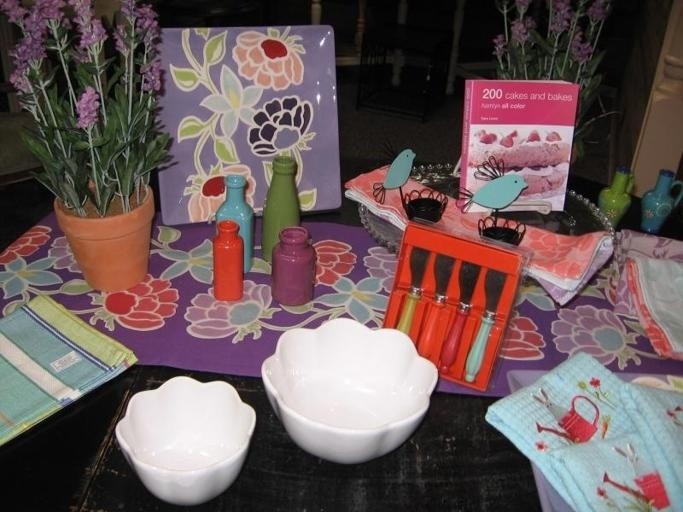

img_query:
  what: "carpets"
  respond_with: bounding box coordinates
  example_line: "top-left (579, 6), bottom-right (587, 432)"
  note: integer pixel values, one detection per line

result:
top-left (0, 208), bottom-right (683, 402)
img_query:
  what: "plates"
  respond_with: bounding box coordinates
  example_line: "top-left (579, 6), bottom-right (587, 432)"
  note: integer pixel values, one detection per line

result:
top-left (361, 160), bottom-right (616, 291)
top-left (145, 24), bottom-right (342, 228)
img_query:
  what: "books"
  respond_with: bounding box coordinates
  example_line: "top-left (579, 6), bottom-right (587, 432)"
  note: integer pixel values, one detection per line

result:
top-left (459, 79), bottom-right (579, 211)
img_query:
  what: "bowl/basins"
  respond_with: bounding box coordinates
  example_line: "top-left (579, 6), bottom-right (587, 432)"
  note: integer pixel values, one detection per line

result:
top-left (260, 318), bottom-right (439, 466)
top-left (110, 375), bottom-right (257, 507)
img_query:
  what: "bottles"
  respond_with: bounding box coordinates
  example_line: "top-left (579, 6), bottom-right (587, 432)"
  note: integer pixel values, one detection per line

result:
top-left (208, 158), bottom-right (316, 305)
top-left (598, 163), bottom-right (682, 234)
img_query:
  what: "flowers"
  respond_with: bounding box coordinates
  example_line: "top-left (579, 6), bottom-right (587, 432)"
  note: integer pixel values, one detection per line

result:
top-left (491, 1), bottom-right (627, 151)
top-left (0, 1), bottom-right (175, 217)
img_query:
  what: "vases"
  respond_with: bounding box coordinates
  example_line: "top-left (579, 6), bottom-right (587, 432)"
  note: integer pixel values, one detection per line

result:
top-left (51, 178), bottom-right (157, 293)
